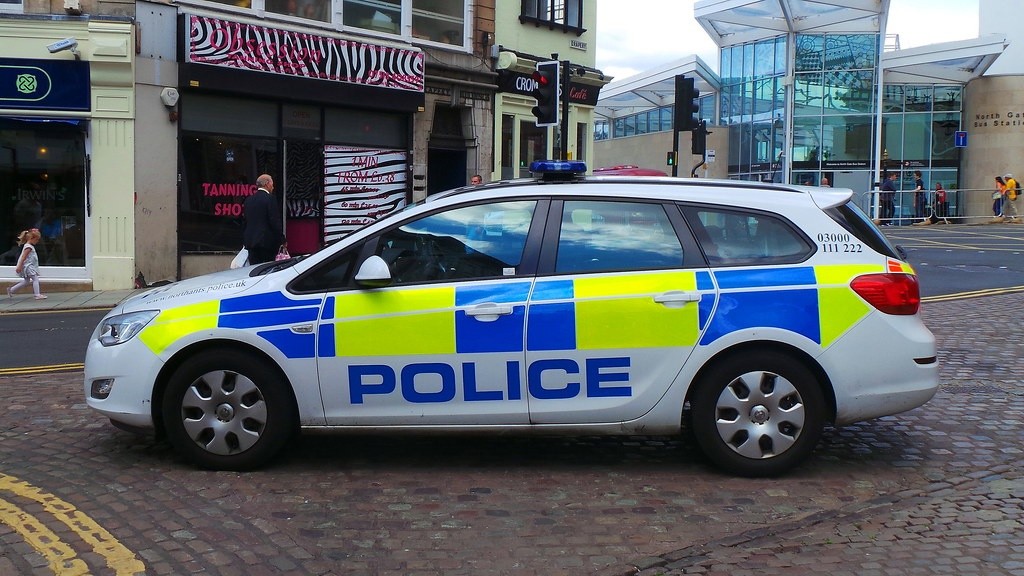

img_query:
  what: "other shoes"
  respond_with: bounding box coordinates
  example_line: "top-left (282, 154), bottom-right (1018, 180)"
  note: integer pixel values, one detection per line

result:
top-left (35, 295), bottom-right (47, 300)
top-left (7, 287), bottom-right (13, 298)
top-left (886, 222), bottom-right (894, 226)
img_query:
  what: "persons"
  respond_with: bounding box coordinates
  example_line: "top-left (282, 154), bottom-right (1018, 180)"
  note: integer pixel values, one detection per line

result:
top-left (880, 173), bottom-right (897, 226)
top-left (992, 174), bottom-right (1018, 218)
top-left (932, 183), bottom-right (946, 222)
top-left (241, 174), bottom-right (288, 265)
top-left (0, 184), bottom-right (67, 263)
top-left (7, 229), bottom-right (47, 299)
top-left (471, 175), bottom-right (482, 185)
top-left (910, 171), bottom-right (926, 222)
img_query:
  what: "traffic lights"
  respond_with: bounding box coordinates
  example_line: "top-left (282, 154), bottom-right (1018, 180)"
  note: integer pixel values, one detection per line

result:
top-left (666, 151), bottom-right (677, 166)
top-left (679, 77), bottom-right (699, 132)
top-left (531, 61), bottom-right (559, 127)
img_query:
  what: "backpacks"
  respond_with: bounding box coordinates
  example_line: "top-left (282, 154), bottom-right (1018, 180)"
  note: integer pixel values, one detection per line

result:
top-left (1014, 179), bottom-right (1022, 195)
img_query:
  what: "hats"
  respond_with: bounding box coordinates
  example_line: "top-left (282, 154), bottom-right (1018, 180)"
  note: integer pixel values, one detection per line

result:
top-left (1003, 174), bottom-right (1012, 179)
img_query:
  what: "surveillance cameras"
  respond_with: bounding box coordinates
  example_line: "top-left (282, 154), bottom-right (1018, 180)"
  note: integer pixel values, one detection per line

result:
top-left (46, 37), bottom-right (77, 54)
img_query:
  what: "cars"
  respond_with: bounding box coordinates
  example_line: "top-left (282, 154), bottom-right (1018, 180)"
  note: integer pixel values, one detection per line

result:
top-left (84, 159), bottom-right (941, 479)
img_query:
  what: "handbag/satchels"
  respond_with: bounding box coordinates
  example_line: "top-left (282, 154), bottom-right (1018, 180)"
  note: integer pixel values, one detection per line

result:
top-left (230, 246), bottom-right (250, 269)
top-left (993, 191), bottom-right (1002, 199)
top-left (275, 245), bottom-right (291, 261)
